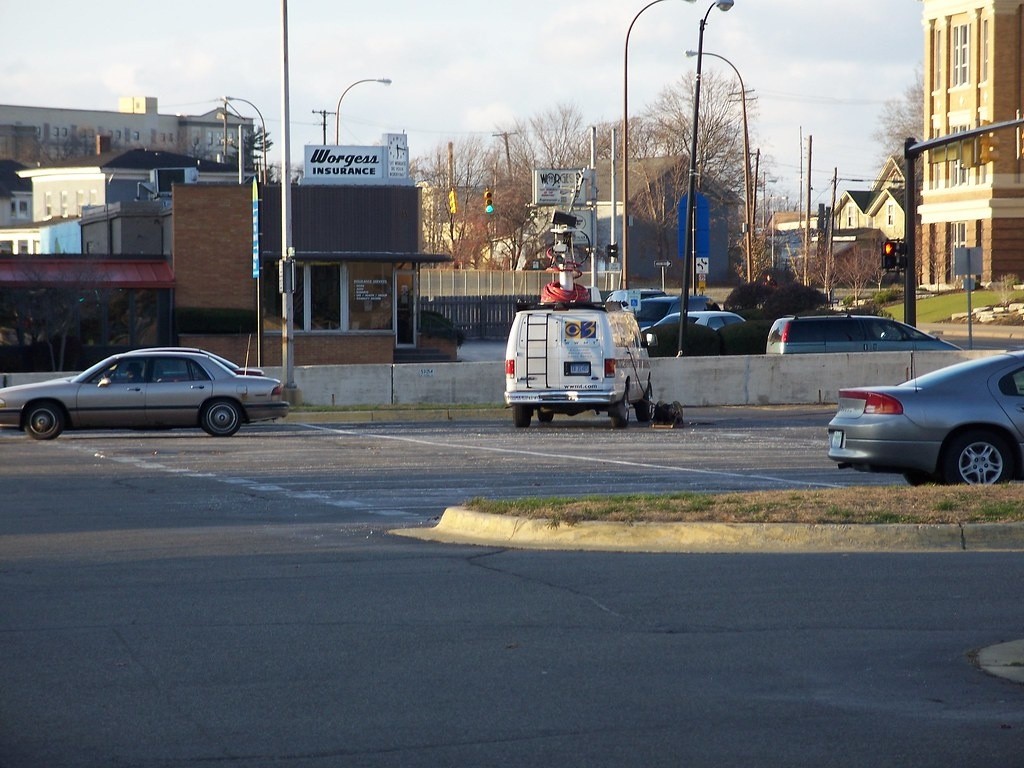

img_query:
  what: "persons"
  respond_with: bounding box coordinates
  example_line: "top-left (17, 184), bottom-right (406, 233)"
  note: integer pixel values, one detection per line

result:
top-left (704, 299), bottom-right (722, 311)
top-left (126, 363), bottom-right (144, 382)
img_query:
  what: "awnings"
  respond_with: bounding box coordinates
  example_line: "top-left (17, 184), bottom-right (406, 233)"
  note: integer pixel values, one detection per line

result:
top-left (261, 251), bottom-right (455, 263)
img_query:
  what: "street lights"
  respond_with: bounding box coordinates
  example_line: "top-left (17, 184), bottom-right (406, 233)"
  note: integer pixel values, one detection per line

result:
top-left (221, 95), bottom-right (266, 186)
top-left (683, 49), bottom-right (754, 285)
top-left (621, 0), bottom-right (699, 289)
top-left (676, 0), bottom-right (737, 356)
top-left (335, 76), bottom-right (393, 145)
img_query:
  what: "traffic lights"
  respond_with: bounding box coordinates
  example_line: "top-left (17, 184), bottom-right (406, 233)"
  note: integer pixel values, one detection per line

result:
top-left (449, 190), bottom-right (459, 213)
top-left (881, 241), bottom-right (897, 271)
top-left (485, 191), bottom-right (493, 214)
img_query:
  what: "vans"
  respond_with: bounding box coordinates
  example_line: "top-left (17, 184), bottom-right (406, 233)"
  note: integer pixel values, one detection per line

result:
top-left (603, 288), bottom-right (668, 310)
top-left (504, 303), bottom-right (656, 428)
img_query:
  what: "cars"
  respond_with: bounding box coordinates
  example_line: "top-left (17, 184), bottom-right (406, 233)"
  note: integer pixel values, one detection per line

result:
top-left (642, 311), bottom-right (747, 333)
top-left (0, 352), bottom-right (292, 437)
top-left (826, 349), bottom-right (1023, 488)
top-left (122, 347), bottom-right (266, 385)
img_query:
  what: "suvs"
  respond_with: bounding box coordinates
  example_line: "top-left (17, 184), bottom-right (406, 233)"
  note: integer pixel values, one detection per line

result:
top-left (633, 295), bottom-right (721, 331)
top-left (764, 312), bottom-right (966, 354)
